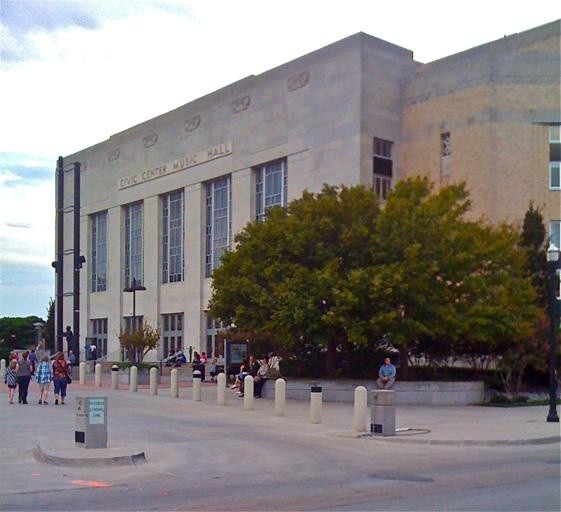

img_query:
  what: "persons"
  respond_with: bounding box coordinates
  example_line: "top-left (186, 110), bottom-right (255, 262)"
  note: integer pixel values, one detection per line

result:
top-left (166, 348), bottom-right (287, 400)
top-left (376, 357), bottom-right (397, 389)
top-left (5, 347), bottom-right (78, 406)
top-left (57, 325), bottom-right (74, 353)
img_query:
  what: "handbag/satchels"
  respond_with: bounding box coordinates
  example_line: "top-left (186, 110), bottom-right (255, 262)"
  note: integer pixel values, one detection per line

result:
top-left (64, 371), bottom-right (71, 384)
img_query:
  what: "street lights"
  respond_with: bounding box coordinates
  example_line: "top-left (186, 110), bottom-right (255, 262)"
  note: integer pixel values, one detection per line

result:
top-left (545, 244), bottom-right (561, 422)
top-left (1, 334), bottom-right (17, 367)
top-left (123, 275), bottom-right (148, 337)
